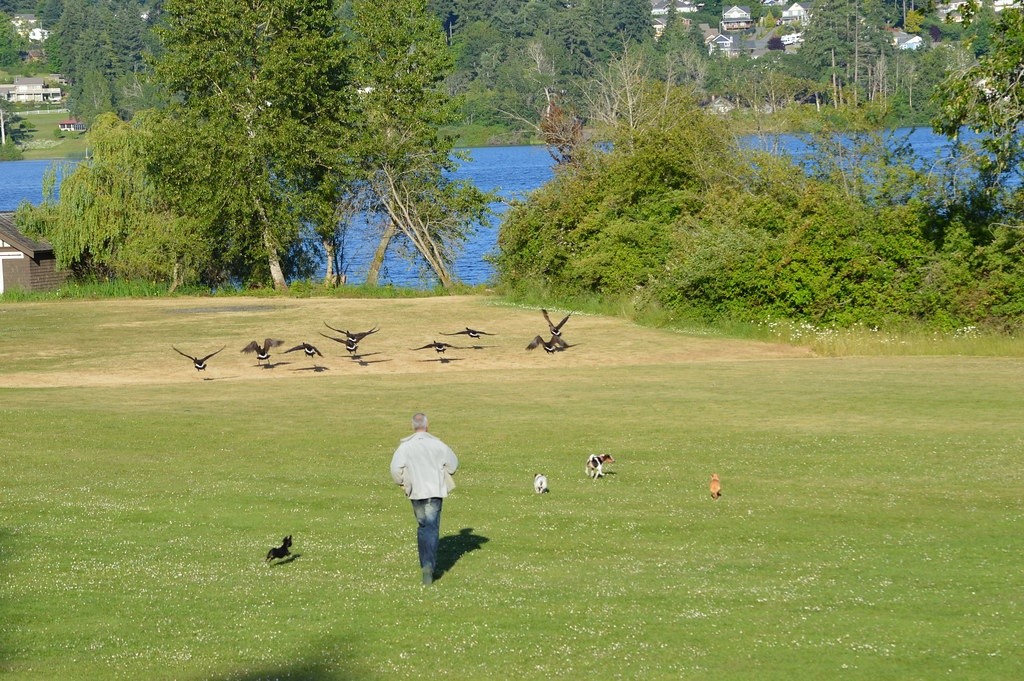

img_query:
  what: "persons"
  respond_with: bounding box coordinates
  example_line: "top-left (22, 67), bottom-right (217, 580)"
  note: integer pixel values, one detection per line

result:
top-left (389, 413), bottom-right (459, 585)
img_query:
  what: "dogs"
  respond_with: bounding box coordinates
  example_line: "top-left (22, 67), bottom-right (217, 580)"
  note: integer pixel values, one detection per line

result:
top-left (534, 474), bottom-right (547, 493)
top-left (585, 454), bottom-right (615, 479)
top-left (710, 473), bottom-right (721, 499)
top-left (265, 534), bottom-right (292, 564)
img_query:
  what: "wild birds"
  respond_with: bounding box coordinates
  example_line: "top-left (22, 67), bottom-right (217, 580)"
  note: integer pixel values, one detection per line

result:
top-left (319, 322), bottom-right (381, 353)
top-left (440, 328), bottom-right (498, 339)
top-left (542, 309), bottom-right (573, 337)
top-left (241, 338), bottom-right (284, 366)
top-left (410, 340), bottom-right (463, 354)
top-left (526, 336), bottom-right (567, 354)
top-left (279, 343), bottom-right (324, 359)
top-left (171, 346), bottom-right (227, 370)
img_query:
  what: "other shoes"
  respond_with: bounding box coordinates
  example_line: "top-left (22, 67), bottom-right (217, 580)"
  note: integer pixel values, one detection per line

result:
top-left (422, 567), bottom-right (433, 585)
